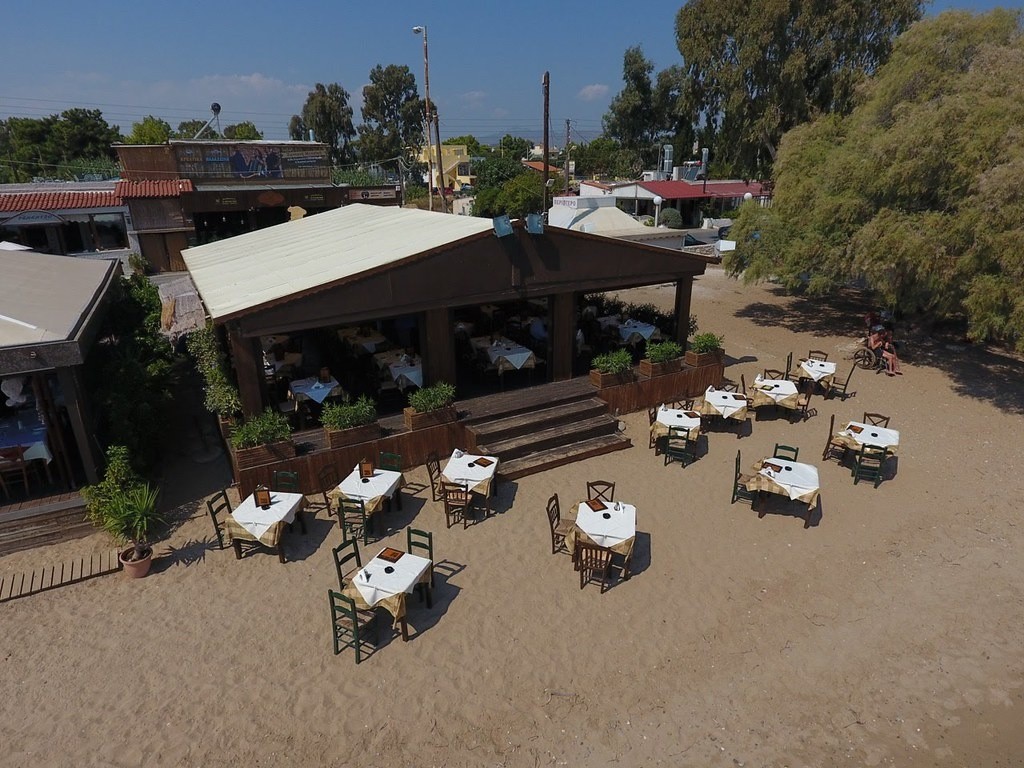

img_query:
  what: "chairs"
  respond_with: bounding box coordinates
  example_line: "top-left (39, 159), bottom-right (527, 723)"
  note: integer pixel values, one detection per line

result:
top-left (204, 348), bottom-right (901, 664)
top-left (265, 365), bottom-right (279, 386)
top-left (270, 394), bottom-right (300, 428)
top-left (0, 443), bottom-right (34, 500)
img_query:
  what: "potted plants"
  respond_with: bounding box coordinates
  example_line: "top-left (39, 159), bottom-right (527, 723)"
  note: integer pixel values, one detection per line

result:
top-left (79, 382), bottom-right (459, 579)
top-left (686, 334), bottom-right (724, 364)
top-left (640, 342), bottom-right (681, 376)
top-left (590, 350), bottom-right (635, 388)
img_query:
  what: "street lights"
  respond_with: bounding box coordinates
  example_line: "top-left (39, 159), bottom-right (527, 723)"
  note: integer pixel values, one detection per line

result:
top-left (653, 195), bottom-right (662, 227)
top-left (412, 24), bottom-right (435, 212)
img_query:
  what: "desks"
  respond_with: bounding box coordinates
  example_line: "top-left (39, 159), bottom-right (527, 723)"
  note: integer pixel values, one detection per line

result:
top-left (656, 407), bottom-right (702, 462)
top-left (703, 388), bottom-right (746, 439)
top-left (0, 412), bottom-right (55, 485)
top-left (754, 380), bottom-right (798, 423)
top-left (846, 422), bottom-right (900, 477)
top-left (352, 547), bottom-right (434, 642)
top-left (574, 500), bottom-right (637, 572)
top-left (445, 451), bottom-right (498, 515)
top-left (329, 467), bottom-right (404, 533)
top-left (797, 357), bottom-right (837, 397)
top-left (264, 299), bottom-right (663, 411)
top-left (224, 489), bottom-right (309, 563)
top-left (755, 456), bottom-right (821, 531)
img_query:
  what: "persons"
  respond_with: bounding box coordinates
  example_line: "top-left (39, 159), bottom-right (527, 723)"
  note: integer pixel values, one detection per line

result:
top-left (698, 201), bottom-right (704, 221)
top-left (229, 144), bottom-right (284, 179)
top-left (863, 307), bottom-right (902, 377)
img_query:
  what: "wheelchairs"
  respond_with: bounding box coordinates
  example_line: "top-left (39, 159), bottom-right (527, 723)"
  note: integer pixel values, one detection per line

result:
top-left (853, 337), bottom-right (895, 376)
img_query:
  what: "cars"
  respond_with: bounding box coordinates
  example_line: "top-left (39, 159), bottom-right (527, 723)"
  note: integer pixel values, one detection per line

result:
top-left (461, 183), bottom-right (475, 190)
